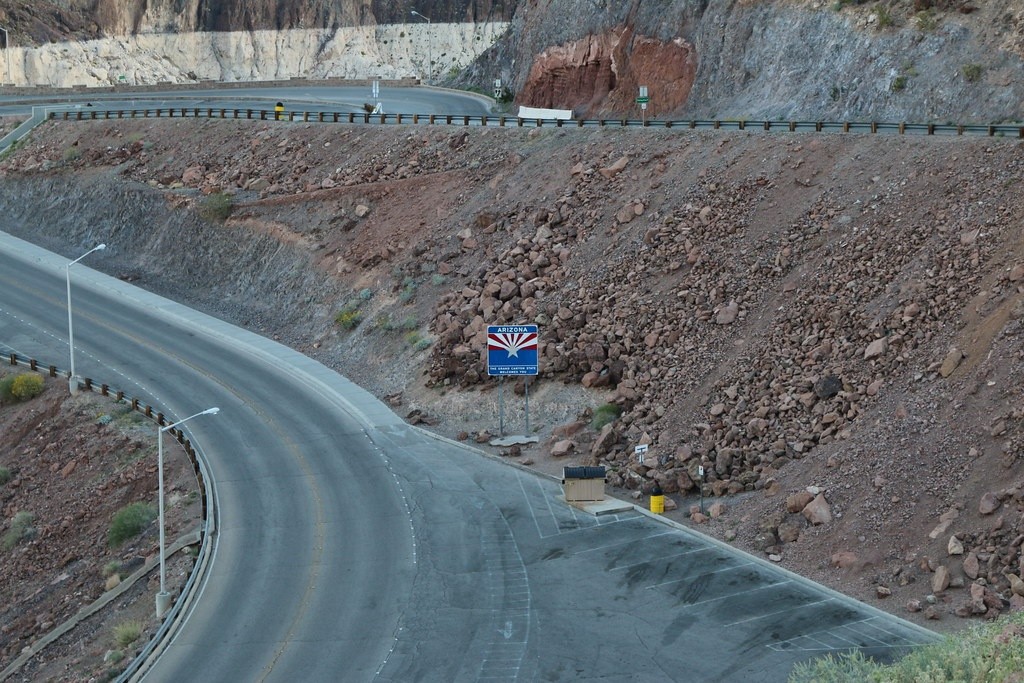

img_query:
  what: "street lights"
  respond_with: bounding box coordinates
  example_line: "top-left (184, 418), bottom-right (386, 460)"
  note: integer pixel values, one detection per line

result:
top-left (0, 27), bottom-right (10, 85)
top-left (412, 11), bottom-right (432, 80)
top-left (65, 240), bottom-right (107, 396)
top-left (153, 405), bottom-right (223, 616)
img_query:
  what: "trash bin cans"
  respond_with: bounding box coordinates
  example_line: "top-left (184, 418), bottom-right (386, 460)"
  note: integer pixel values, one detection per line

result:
top-left (560, 466), bottom-right (608, 502)
top-left (650, 490), bottom-right (665, 514)
